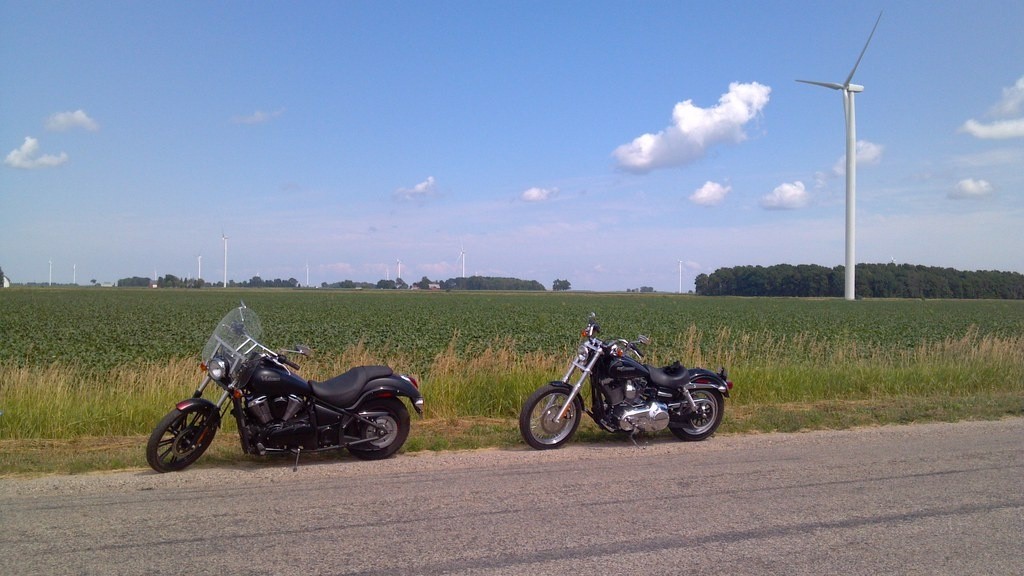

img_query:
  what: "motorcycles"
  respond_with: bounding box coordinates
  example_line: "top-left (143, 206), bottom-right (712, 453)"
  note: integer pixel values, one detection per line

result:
top-left (146, 300), bottom-right (425, 474)
top-left (519, 312), bottom-right (735, 451)
